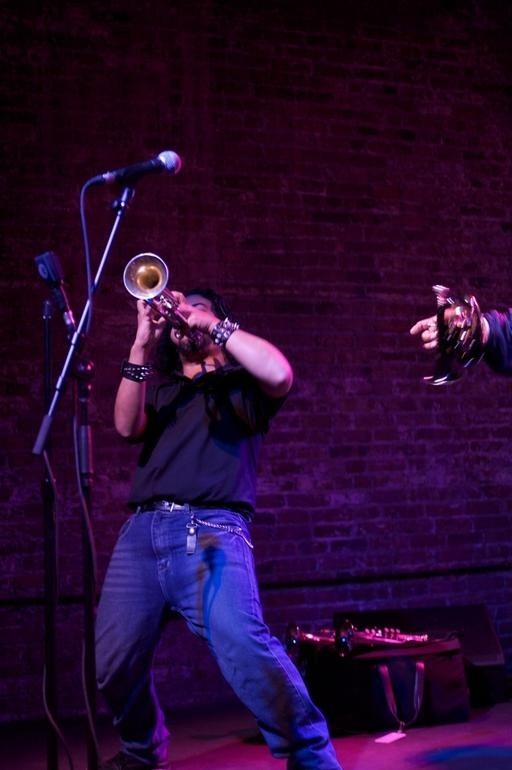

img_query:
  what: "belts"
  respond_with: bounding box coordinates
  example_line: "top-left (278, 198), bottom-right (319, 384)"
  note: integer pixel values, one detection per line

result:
top-left (155, 500), bottom-right (198, 512)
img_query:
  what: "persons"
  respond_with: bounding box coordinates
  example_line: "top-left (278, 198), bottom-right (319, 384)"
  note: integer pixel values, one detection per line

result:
top-left (89, 286), bottom-right (345, 770)
top-left (409, 280), bottom-right (511, 379)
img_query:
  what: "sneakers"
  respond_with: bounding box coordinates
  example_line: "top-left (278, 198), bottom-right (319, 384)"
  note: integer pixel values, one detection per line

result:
top-left (102, 750), bottom-right (170, 770)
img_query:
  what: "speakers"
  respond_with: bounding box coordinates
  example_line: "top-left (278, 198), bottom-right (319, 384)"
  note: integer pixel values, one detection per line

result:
top-left (333, 603), bottom-right (505, 705)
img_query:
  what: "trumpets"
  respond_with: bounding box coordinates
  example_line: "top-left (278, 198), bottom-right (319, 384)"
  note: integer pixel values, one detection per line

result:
top-left (285, 619), bottom-right (429, 659)
top-left (124, 251), bottom-right (198, 343)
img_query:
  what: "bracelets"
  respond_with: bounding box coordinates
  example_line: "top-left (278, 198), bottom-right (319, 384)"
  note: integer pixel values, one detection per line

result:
top-left (121, 361), bottom-right (150, 387)
top-left (207, 316), bottom-right (241, 348)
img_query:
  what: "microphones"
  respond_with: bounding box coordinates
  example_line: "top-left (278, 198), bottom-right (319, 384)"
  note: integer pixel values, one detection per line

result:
top-left (98, 150), bottom-right (184, 185)
top-left (34, 251), bottom-right (75, 338)
